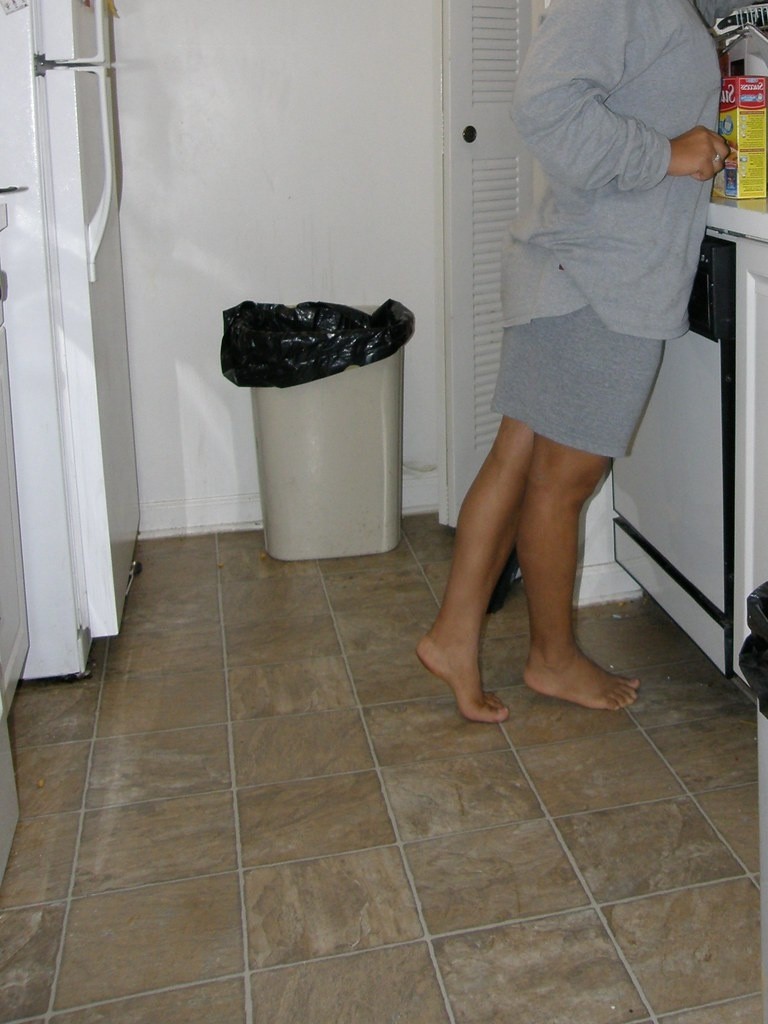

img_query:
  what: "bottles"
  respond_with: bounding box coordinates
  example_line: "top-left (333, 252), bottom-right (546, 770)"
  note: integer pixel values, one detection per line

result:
top-left (723, 162), bottom-right (737, 197)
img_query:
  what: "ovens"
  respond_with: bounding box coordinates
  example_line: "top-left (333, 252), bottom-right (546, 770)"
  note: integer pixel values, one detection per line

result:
top-left (611, 238), bottom-right (736, 679)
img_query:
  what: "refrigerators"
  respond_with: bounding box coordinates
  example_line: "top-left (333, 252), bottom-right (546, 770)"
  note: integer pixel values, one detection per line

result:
top-left (0, 1), bottom-right (141, 679)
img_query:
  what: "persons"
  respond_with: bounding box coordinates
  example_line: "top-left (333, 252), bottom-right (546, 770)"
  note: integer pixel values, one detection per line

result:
top-left (413, 0), bottom-right (762, 724)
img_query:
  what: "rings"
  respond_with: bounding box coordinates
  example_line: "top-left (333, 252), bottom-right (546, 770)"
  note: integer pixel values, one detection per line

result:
top-left (712, 154), bottom-right (720, 163)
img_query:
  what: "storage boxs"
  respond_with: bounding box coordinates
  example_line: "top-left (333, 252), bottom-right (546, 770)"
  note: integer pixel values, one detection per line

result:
top-left (706, 75), bottom-right (766, 200)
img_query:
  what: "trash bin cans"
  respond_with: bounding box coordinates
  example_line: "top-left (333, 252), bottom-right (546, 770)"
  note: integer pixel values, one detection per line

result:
top-left (746, 582), bottom-right (767, 978)
top-left (250, 304), bottom-right (404, 560)
